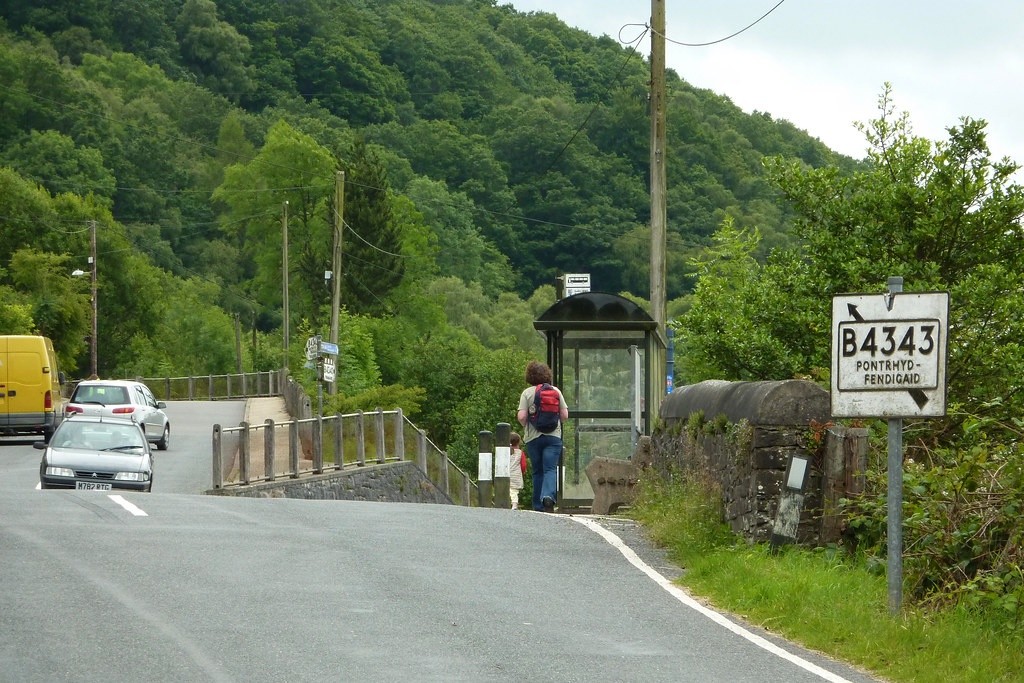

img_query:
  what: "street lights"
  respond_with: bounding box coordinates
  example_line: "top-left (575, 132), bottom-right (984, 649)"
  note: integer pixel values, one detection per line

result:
top-left (71, 219), bottom-right (98, 375)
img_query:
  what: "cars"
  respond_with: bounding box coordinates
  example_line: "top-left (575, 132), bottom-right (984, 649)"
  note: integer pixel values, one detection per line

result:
top-left (33, 410), bottom-right (154, 493)
top-left (66, 380), bottom-right (171, 451)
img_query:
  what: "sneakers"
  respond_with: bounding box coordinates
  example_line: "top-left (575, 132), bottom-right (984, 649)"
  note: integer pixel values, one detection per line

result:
top-left (512, 503), bottom-right (518, 510)
top-left (543, 497), bottom-right (554, 512)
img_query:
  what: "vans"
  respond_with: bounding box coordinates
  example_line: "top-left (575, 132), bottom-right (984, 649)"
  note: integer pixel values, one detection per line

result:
top-left (0, 335), bottom-right (64, 445)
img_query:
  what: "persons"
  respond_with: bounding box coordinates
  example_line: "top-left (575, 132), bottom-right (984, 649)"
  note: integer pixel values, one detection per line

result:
top-left (509, 431), bottom-right (527, 511)
top-left (105, 431), bottom-right (128, 450)
top-left (63, 427), bottom-right (94, 450)
top-left (518, 362), bottom-right (569, 512)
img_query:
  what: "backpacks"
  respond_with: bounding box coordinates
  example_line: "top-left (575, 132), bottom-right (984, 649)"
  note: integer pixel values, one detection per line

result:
top-left (528, 384), bottom-right (560, 433)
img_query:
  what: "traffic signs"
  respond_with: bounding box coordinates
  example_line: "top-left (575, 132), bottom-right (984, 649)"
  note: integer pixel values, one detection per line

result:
top-left (320, 341), bottom-right (339, 355)
top-left (831, 291), bottom-right (951, 419)
top-left (323, 357), bottom-right (336, 382)
top-left (303, 337), bottom-right (319, 361)
